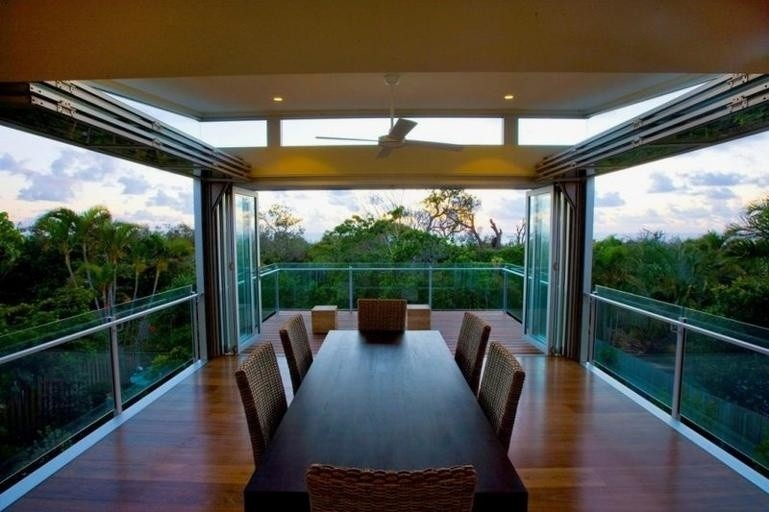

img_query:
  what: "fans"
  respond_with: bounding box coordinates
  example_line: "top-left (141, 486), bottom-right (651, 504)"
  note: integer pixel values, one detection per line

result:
top-left (309, 71), bottom-right (467, 162)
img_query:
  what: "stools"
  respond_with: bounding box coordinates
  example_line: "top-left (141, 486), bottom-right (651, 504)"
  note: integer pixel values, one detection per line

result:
top-left (405, 302), bottom-right (431, 330)
top-left (310, 304), bottom-right (338, 336)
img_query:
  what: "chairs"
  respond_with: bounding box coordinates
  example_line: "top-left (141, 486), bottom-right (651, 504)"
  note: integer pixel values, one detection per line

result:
top-left (475, 339), bottom-right (526, 456)
top-left (232, 339), bottom-right (289, 469)
top-left (355, 297), bottom-right (407, 333)
top-left (302, 460), bottom-right (479, 511)
top-left (277, 310), bottom-right (314, 399)
top-left (453, 310), bottom-right (492, 398)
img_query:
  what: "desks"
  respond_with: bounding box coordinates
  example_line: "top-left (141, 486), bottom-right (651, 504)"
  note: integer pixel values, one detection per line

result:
top-left (241, 328), bottom-right (530, 512)
